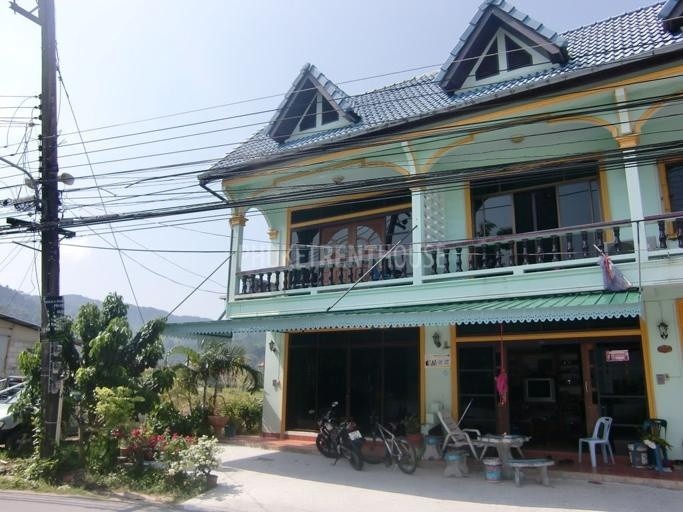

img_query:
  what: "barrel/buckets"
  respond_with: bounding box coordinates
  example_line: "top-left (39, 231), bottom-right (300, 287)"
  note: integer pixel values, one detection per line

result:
top-left (483, 457), bottom-right (503, 480)
top-left (627, 443), bottom-right (648, 469)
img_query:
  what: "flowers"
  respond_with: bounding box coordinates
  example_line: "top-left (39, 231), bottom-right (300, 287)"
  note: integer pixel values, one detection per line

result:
top-left (110, 428), bottom-right (193, 462)
top-left (178, 434), bottom-right (225, 473)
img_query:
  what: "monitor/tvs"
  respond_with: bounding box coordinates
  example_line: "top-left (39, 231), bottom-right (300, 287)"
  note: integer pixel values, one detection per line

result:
top-left (522, 378), bottom-right (556, 402)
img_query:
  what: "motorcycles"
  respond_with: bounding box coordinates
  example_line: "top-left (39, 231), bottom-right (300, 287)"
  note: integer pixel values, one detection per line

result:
top-left (306, 397), bottom-right (366, 472)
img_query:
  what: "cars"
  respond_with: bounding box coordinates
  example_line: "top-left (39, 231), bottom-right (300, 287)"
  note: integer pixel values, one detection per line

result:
top-left (0, 385), bottom-right (34, 448)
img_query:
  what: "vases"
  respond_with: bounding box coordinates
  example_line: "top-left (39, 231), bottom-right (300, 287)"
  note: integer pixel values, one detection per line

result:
top-left (199, 473), bottom-right (218, 489)
top-left (118, 448), bottom-right (164, 462)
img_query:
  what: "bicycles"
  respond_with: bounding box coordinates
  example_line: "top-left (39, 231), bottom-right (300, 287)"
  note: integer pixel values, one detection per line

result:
top-left (361, 409), bottom-right (418, 474)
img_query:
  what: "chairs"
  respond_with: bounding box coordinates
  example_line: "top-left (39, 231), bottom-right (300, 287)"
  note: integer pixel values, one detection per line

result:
top-left (437, 408), bottom-right (531, 480)
top-left (578, 416), bottom-right (616, 467)
top-left (632, 419), bottom-right (668, 472)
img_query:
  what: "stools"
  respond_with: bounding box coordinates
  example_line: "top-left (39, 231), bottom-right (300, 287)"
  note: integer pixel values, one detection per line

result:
top-left (506, 457), bottom-right (554, 487)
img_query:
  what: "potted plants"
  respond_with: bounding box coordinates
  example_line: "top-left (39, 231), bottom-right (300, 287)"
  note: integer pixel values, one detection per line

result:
top-left (400, 412), bottom-right (423, 448)
